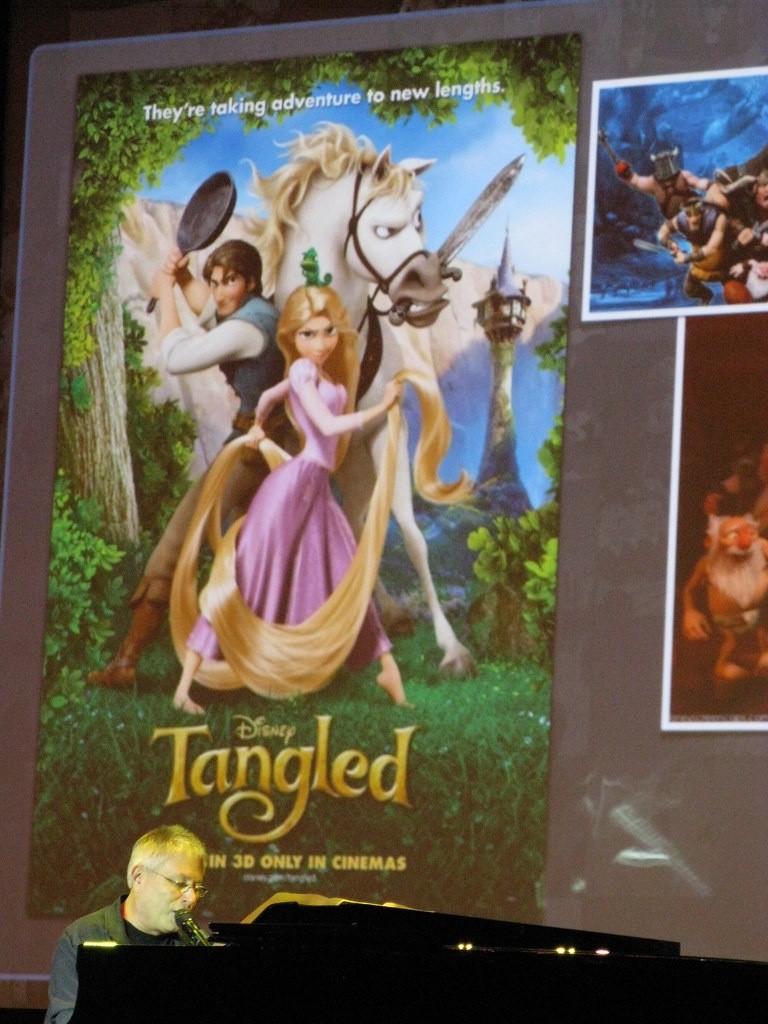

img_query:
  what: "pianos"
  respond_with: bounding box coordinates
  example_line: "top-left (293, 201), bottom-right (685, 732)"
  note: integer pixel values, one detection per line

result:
top-left (65, 901), bottom-right (768, 1024)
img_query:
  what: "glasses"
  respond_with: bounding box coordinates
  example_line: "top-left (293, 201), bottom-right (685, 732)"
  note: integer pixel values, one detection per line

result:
top-left (144, 867), bottom-right (207, 898)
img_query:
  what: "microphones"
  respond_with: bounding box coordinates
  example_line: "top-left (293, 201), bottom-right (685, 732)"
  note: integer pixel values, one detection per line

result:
top-left (174, 909), bottom-right (212, 946)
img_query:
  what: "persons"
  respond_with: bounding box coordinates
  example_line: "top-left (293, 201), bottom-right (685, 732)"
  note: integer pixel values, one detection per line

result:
top-left (44, 823), bottom-right (210, 1024)
